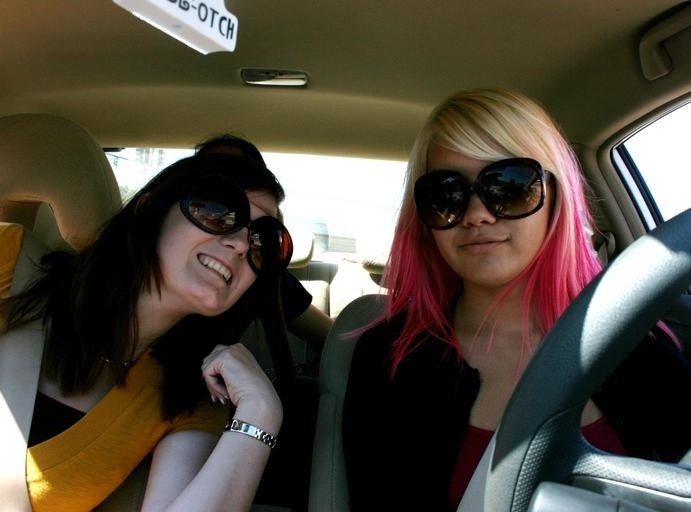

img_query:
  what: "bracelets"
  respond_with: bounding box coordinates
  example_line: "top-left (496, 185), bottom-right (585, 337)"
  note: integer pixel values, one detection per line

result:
top-left (224, 416), bottom-right (277, 449)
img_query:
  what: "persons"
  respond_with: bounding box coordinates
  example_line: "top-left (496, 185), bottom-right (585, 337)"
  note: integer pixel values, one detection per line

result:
top-left (0, 135), bottom-right (286, 511)
top-left (188, 136), bottom-right (333, 341)
top-left (344, 83), bottom-right (689, 512)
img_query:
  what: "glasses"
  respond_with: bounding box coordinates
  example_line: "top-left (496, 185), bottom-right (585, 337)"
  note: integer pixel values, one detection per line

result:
top-left (411, 157), bottom-right (548, 231)
top-left (178, 179), bottom-right (293, 281)
